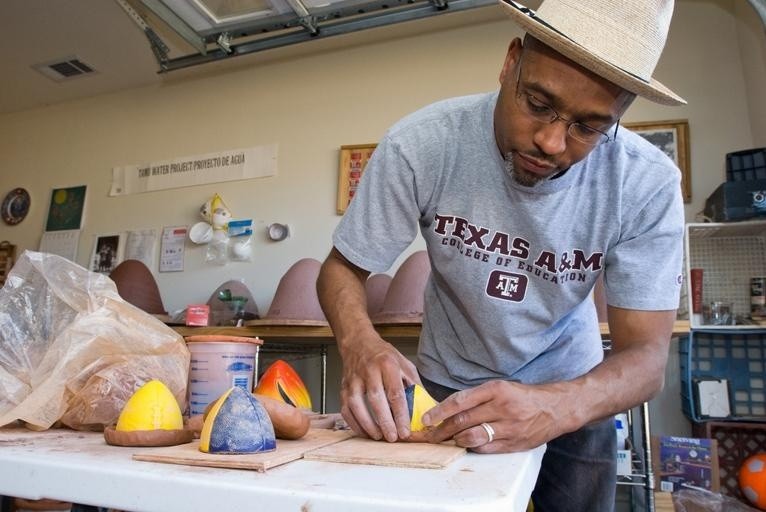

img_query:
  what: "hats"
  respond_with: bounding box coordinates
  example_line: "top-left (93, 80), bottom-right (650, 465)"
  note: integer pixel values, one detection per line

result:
top-left (498, 0), bottom-right (688, 108)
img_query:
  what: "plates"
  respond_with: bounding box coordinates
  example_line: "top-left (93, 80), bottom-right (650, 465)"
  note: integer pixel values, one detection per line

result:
top-left (1, 187), bottom-right (30, 225)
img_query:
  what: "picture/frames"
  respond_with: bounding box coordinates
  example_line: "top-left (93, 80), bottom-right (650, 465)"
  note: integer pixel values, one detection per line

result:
top-left (619, 118), bottom-right (692, 204)
top-left (337, 143), bottom-right (377, 215)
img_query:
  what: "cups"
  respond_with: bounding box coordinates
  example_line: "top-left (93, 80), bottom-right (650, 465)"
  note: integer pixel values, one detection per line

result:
top-left (702, 301), bottom-right (734, 325)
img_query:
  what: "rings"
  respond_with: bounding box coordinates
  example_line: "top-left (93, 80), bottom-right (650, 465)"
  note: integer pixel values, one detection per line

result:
top-left (482, 419), bottom-right (498, 442)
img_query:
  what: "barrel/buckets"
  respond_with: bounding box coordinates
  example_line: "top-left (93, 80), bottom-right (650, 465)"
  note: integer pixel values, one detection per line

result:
top-left (186, 340), bottom-right (258, 420)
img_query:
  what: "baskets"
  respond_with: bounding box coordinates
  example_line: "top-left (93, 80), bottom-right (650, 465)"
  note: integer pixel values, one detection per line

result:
top-left (679, 326), bottom-right (766, 422)
top-left (684, 218), bottom-right (762, 329)
top-left (692, 421), bottom-right (765, 503)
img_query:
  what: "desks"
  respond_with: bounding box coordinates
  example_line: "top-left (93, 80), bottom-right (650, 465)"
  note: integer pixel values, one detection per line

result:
top-left (0, 417), bottom-right (547, 512)
top-left (172, 320), bottom-right (692, 512)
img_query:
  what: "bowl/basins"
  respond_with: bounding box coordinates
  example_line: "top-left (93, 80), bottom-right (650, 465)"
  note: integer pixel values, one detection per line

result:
top-left (212, 300), bottom-right (244, 322)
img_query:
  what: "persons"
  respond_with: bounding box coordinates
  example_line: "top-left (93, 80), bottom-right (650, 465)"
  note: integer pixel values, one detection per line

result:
top-left (319, 1), bottom-right (686, 511)
top-left (105, 245), bottom-right (112, 272)
top-left (99, 243), bottom-right (108, 272)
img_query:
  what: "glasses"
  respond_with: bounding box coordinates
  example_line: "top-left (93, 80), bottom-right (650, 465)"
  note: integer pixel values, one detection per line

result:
top-left (514, 58), bottom-right (620, 146)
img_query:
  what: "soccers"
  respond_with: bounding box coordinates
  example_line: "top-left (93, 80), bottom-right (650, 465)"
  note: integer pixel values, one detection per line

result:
top-left (739, 454), bottom-right (766, 510)
